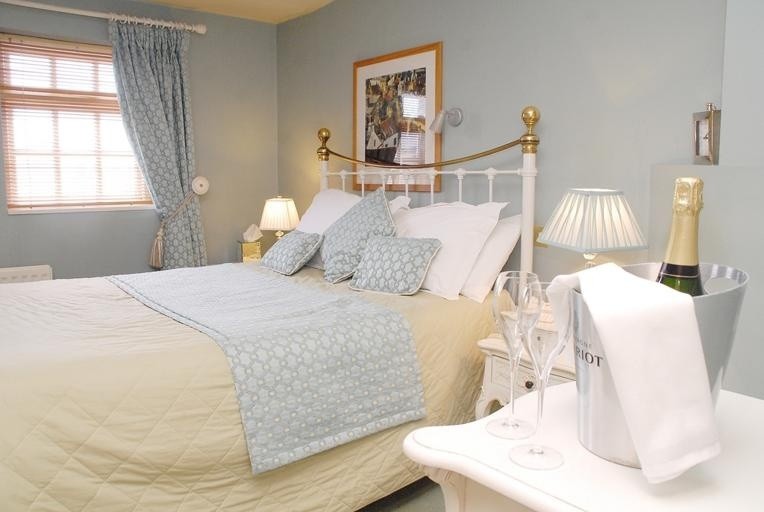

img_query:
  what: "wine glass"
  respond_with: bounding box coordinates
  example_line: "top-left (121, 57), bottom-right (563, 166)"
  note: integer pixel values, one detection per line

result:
top-left (486, 269), bottom-right (572, 470)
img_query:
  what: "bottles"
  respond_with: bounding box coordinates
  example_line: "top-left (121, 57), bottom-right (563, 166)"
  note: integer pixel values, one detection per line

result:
top-left (656, 176), bottom-right (704, 296)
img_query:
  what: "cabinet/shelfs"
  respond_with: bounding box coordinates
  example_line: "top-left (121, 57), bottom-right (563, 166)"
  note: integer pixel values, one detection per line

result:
top-left (402, 381), bottom-right (763, 512)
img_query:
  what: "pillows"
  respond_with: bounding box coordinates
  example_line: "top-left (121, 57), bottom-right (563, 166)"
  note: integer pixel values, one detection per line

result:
top-left (259, 188), bottom-right (522, 305)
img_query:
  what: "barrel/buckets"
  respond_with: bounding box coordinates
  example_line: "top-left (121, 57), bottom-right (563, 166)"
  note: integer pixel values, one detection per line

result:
top-left (571, 263), bottom-right (748, 470)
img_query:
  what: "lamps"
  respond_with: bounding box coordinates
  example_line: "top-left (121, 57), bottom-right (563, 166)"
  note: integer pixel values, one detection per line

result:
top-left (536, 188), bottom-right (651, 272)
top-left (430, 107), bottom-right (462, 135)
top-left (258, 195), bottom-right (301, 240)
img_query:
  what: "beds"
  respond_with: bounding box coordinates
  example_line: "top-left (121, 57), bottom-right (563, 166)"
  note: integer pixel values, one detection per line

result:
top-left (0, 105), bottom-right (540, 512)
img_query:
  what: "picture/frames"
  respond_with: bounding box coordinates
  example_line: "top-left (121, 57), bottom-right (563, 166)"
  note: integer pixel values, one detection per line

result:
top-left (352, 40), bottom-right (444, 192)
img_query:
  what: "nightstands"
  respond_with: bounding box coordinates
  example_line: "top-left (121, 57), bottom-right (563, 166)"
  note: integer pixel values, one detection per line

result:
top-left (474, 303), bottom-right (575, 426)
